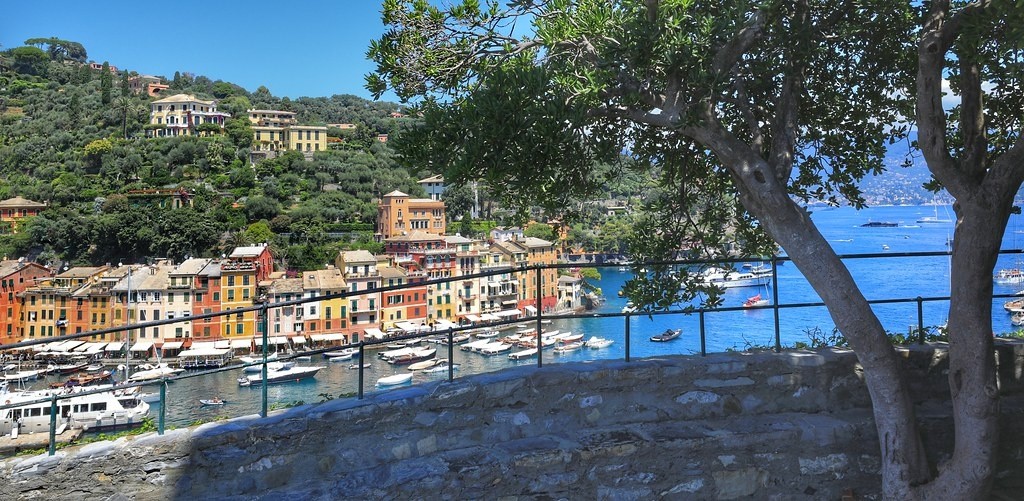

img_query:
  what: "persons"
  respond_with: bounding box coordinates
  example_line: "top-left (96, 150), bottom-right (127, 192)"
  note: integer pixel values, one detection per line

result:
top-left (213, 396), bottom-right (218, 403)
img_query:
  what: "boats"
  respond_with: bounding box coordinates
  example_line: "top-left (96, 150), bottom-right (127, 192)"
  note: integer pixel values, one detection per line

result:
top-left (915, 178), bottom-right (954, 224)
top-left (421, 363), bottom-right (461, 376)
top-left (740, 260), bottom-right (771, 307)
top-left (669, 262), bottom-right (774, 291)
top-left (475, 328), bottom-right (500, 338)
top-left (905, 223), bottom-right (922, 229)
top-left (649, 328), bottom-right (683, 341)
top-left (349, 363), bottom-right (372, 370)
top-left (622, 299), bottom-right (639, 313)
top-left (406, 356), bottom-right (449, 371)
top-left (992, 264), bottom-right (1024, 285)
top-left (1003, 290), bottom-right (1024, 327)
top-left (507, 347), bottom-right (538, 361)
top-left (860, 220), bottom-right (898, 228)
top-left (462, 318), bottom-right (614, 354)
top-left (329, 353), bottom-right (353, 363)
top-left (0, 266), bottom-right (327, 433)
top-left (379, 337), bottom-right (439, 368)
top-left (374, 372), bottom-right (414, 389)
top-left (198, 396), bottom-right (227, 405)
top-left (441, 333), bottom-right (471, 343)
top-left (321, 349), bottom-right (360, 360)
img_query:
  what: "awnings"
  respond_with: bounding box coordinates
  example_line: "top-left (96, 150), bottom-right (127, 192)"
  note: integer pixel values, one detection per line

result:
top-left (310, 333), bottom-right (345, 342)
top-left (130, 340), bottom-right (156, 352)
top-left (161, 341), bottom-right (184, 350)
top-left (364, 328), bottom-right (384, 340)
top-left (10, 337), bottom-right (125, 357)
top-left (465, 309), bottom-right (522, 321)
top-left (488, 281), bottom-right (501, 287)
top-left (189, 338), bottom-right (251, 349)
top-left (254, 335), bottom-right (307, 346)
top-left (502, 299), bottom-right (518, 305)
top-left (394, 321), bottom-right (415, 333)
